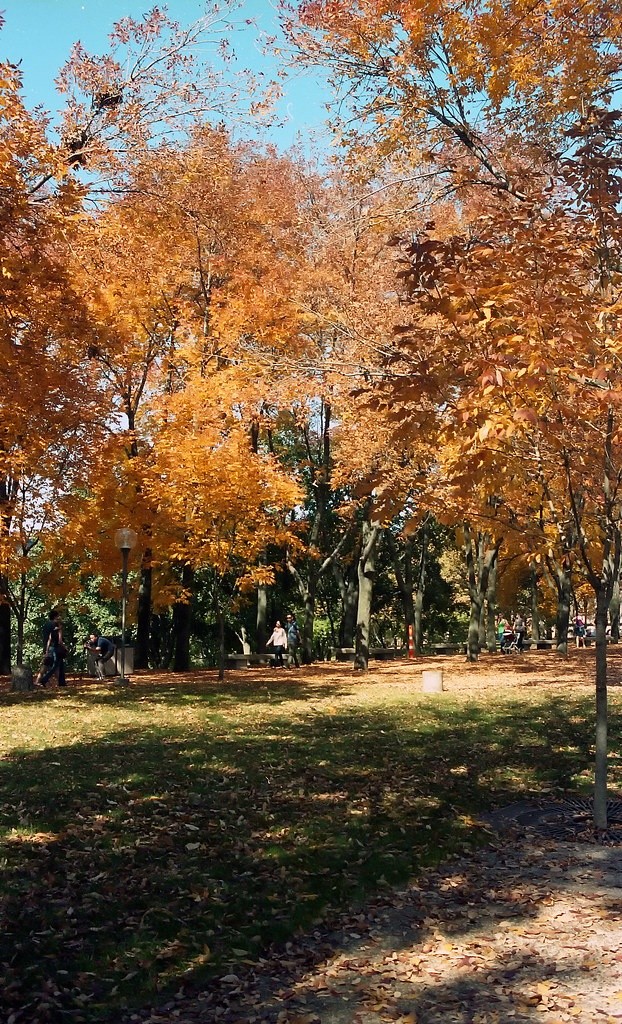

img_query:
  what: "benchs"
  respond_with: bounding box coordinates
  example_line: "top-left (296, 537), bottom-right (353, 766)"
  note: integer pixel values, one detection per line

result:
top-left (585, 636), bottom-right (615, 648)
top-left (431, 642), bottom-right (468, 655)
top-left (225, 654), bottom-right (288, 670)
top-left (335, 648), bottom-right (395, 663)
top-left (521, 639), bottom-right (557, 650)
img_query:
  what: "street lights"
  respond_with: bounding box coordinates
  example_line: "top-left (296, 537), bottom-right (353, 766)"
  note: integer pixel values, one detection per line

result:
top-left (114, 527), bottom-right (137, 688)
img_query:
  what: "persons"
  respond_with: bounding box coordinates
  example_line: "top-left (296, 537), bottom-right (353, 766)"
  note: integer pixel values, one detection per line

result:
top-left (83, 633), bottom-right (115, 681)
top-left (513, 612), bottom-right (526, 652)
top-left (265, 619), bottom-right (288, 668)
top-left (34, 610), bottom-right (59, 686)
top-left (39, 616), bottom-right (67, 687)
top-left (494, 611), bottom-right (516, 653)
top-left (572, 610), bottom-right (587, 649)
top-left (284, 613), bottom-right (303, 669)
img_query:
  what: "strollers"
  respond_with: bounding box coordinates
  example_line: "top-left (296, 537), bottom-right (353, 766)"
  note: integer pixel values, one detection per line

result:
top-left (502, 628), bottom-right (524, 655)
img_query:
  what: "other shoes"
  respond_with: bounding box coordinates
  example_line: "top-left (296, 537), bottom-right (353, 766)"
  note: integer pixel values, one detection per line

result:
top-left (293, 665), bottom-right (300, 668)
top-left (34, 683), bottom-right (47, 689)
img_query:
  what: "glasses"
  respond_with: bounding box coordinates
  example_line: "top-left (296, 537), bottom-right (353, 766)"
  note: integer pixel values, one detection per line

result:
top-left (287, 617), bottom-right (292, 619)
